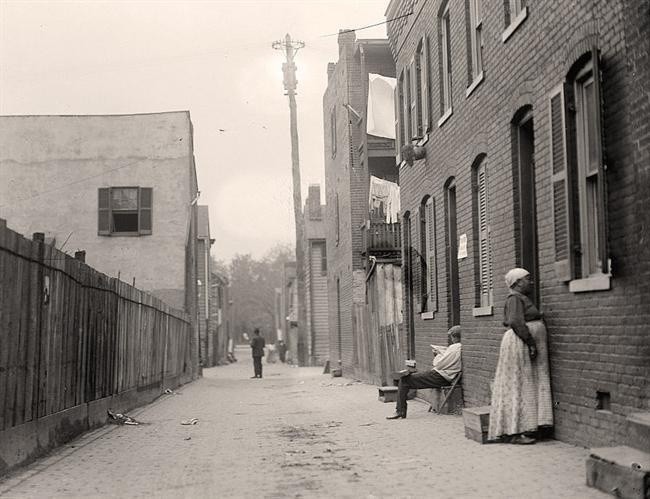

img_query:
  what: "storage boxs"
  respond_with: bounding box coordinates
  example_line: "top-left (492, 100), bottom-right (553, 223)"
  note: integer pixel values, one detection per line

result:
top-left (377, 386), bottom-right (398, 403)
top-left (462, 405), bottom-right (491, 432)
top-left (464, 426), bottom-right (504, 444)
top-left (585, 444), bottom-right (650, 499)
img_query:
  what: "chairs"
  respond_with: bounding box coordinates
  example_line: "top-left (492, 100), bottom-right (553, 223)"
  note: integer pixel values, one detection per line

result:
top-left (427, 371), bottom-right (463, 415)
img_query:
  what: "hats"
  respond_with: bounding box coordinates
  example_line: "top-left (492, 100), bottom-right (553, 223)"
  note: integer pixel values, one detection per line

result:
top-left (504, 267), bottom-right (529, 287)
top-left (446, 325), bottom-right (460, 334)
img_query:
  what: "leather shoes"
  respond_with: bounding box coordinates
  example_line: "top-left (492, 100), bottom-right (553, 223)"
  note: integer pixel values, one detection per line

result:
top-left (512, 437), bottom-right (536, 446)
top-left (386, 411), bottom-right (406, 419)
top-left (389, 369), bottom-right (410, 380)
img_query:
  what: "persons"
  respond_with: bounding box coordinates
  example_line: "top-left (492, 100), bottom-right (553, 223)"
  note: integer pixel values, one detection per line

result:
top-left (486, 268), bottom-right (553, 445)
top-left (247, 327), bottom-right (265, 380)
top-left (386, 324), bottom-right (462, 418)
